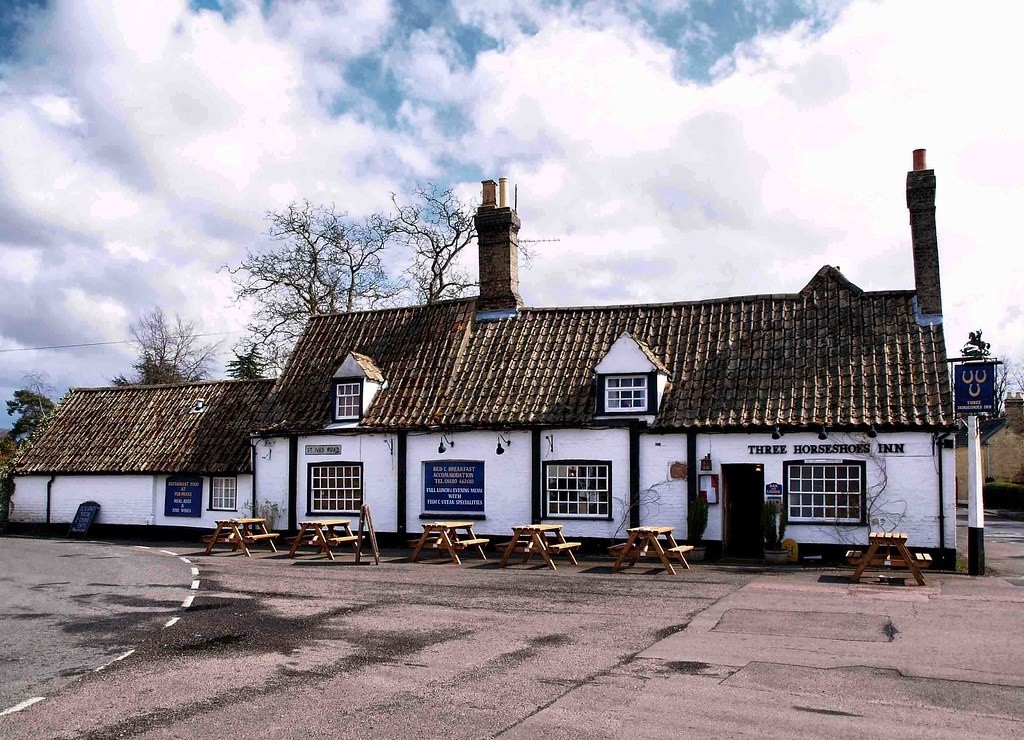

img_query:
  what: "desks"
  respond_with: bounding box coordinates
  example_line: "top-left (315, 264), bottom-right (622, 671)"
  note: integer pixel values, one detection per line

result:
top-left (205, 518), bottom-right (277, 557)
top-left (611, 526), bottom-right (689, 575)
top-left (500, 524), bottom-right (577, 571)
top-left (409, 522), bottom-right (487, 564)
top-left (288, 520), bottom-right (362, 560)
top-left (851, 532), bottom-right (926, 586)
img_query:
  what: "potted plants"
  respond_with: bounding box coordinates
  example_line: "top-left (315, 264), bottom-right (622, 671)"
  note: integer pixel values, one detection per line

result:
top-left (238, 497), bottom-right (285, 547)
top-left (683, 496), bottom-right (709, 562)
top-left (761, 501), bottom-right (789, 564)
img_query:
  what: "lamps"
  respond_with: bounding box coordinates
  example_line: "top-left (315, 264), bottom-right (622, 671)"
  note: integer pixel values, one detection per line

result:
top-left (771, 428), bottom-right (784, 440)
top-left (868, 426), bottom-right (878, 438)
top-left (818, 427), bottom-right (828, 440)
top-left (700, 453), bottom-right (713, 471)
top-left (495, 434), bottom-right (511, 455)
top-left (438, 434), bottom-right (454, 453)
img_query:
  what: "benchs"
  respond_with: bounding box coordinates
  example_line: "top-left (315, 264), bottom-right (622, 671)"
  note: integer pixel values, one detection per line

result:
top-left (846, 550), bottom-right (862, 559)
top-left (607, 543), bottom-right (636, 551)
top-left (496, 541), bottom-right (581, 551)
top-left (202, 532), bottom-right (280, 540)
top-left (916, 552), bottom-right (933, 561)
top-left (408, 537), bottom-right (489, 545)
top-left (668, 545), bottom-right (694, 554)
top-left (285, 534), bottom-right (366, 543)
